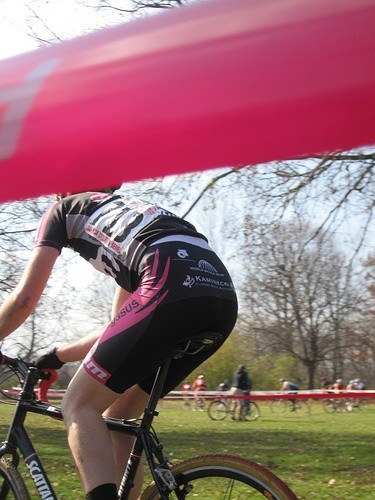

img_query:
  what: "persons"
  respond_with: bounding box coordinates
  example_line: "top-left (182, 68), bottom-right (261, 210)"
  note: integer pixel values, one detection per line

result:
top-left (2, 181), bottom-right (239, 500)
top-left (180, 374), bottom-right (374, 409)
top-left (242, 376), bottom-right (252, 417)
top-left (228, 365), bottom-right (248, 421)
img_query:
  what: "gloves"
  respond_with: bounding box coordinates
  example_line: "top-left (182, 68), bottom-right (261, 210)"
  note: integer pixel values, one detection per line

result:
top-left (35, 347), bottom-right (65, 381)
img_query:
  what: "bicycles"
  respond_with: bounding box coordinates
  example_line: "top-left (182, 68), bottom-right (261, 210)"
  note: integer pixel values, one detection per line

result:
top-left (268, 395), bottom-right (308, 414)
top-left (0, 330), bottom-right (291, 500)
top-left (322, 396), bottom-right (367, 414)
top-left (207, 392), bottom-right (261, 421)
